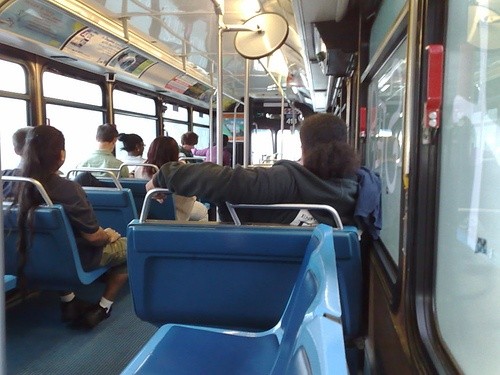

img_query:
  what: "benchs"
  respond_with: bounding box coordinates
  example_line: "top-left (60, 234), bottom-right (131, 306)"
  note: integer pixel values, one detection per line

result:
top-left (0, 176), bottom-right (113, 321)
top-left (119, 223), bottom-right (350, 375)
top-left (125, 188), bottom-right (365, 342)
top-left (119, 223), bottom-right (349, 375)
top-left (66, 170), bottom-right (138, 237)
top-left (69, 163), bottom-right (160, 220)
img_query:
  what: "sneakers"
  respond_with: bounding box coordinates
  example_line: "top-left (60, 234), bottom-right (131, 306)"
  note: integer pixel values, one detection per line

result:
top-left (61, 296), bottom-right (94, 323)
top-left (82, 300), bottom-right (112, 328)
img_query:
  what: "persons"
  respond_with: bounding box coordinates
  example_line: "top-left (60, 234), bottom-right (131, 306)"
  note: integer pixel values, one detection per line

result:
top-left (134, 136), bottom-right (180, 180)
top-left (11, 125), bottom-right (128, 329)
top-left (0, 126), bottom-right (110, 202)
top-left (191, 134), bottom-right (231, 166)
top-left (117, 133), bottom-right (146, 174)
top-left (145, 112), bottom-right (382, 252)
top-left (177, 131), bottom-right (199, 163)
top-left (73, 123), bottom-right (129, 178)
